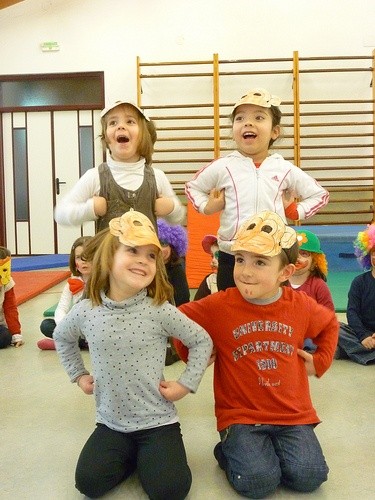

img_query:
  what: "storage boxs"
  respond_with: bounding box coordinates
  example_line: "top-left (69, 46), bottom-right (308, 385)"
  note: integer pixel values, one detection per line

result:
top-left (308, 229), bottom-right (367, 270)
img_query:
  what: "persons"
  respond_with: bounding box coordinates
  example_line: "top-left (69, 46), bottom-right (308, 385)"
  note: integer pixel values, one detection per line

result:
top-left (156, 218), bottom-right (191, 307)
top-left (283, 229), bottom-right (335, 311)
top-left (53, 208), bottom-right (213, 500)
top-left (0, 246), bottom-right (24, 348)
top-left (194, 235), bottom-right (219, 301)
top-left (334, 221), bottom-right (375, 366)
top-left (184, 90), bottom-right (330, 292)
top-left (37, 236), bottom-right (94, 350)
top-left (54, 101), bottom-right (186, 235)
top-left (173, 212), bottom-right (328, 496)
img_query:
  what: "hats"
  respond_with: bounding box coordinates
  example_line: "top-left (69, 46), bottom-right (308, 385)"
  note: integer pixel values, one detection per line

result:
top-left (202, 235), bottom-right (217, 254)
top-left (295, 229), bottom-right (324, 255)
